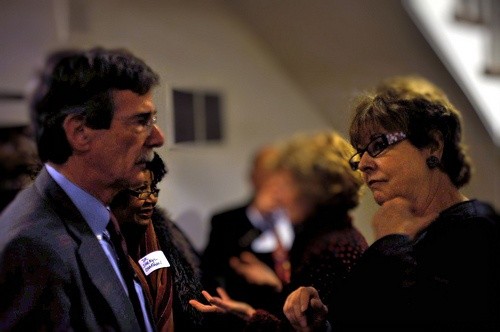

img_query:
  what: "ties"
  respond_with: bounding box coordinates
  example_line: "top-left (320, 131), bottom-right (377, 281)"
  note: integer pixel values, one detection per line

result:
top-left (105, 209), bottom-right (149, 332)
top-left (265, 218), bottom-right (291, 284)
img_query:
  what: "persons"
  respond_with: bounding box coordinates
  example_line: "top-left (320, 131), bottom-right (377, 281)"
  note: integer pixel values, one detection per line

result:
top-left (200, 141), bottom-right (291, 332)
top-left (282, 75), bottom-right (500, 332)
top-left (108, 152), bottom-right (200, 332)
top-left (0, 45), bottom-right (161, 332)
top-left (189, 130), bottom-right (370, 332)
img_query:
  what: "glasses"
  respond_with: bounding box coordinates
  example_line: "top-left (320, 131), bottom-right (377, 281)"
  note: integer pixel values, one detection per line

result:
top-left (348, 129), bottom-right (407, 171)
top-left (124, 187), bottom-right (161, 200)
